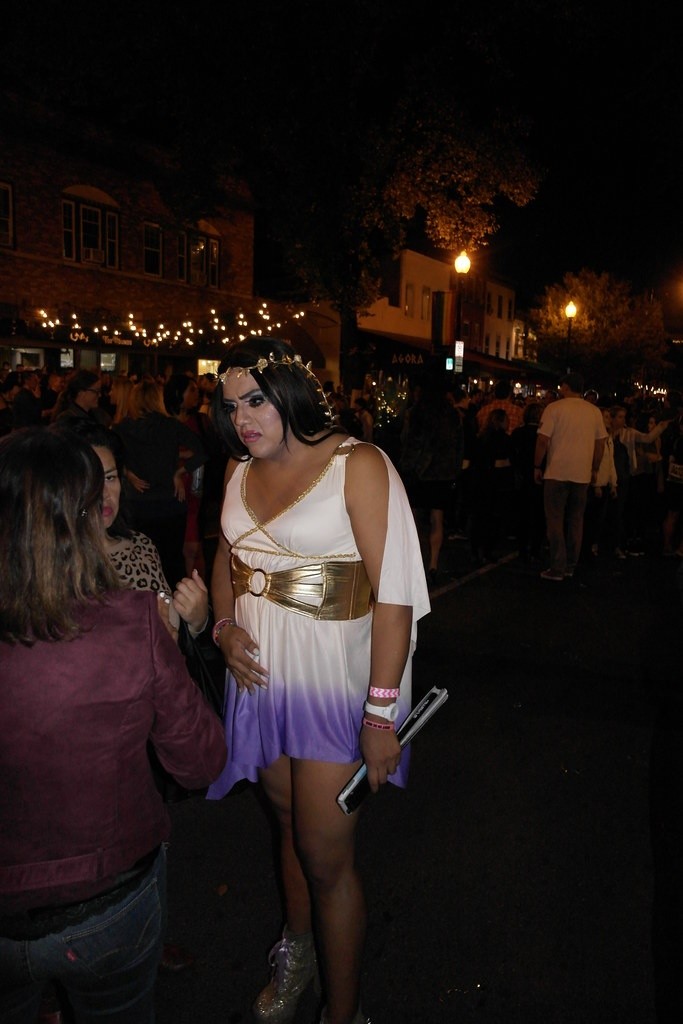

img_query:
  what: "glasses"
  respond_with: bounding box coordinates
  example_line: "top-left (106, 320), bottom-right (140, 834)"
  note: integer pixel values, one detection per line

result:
top-left (84, 388), bottom-right (101, 395)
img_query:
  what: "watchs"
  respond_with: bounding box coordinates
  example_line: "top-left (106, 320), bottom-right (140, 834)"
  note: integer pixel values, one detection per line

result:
top-left (364, 700), bottom-right (400, 722)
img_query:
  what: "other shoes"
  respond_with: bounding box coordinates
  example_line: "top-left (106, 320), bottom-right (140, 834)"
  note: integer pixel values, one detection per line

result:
top-left (540, 569), bottom-right (564, 580)
top-left (564, 569), bottom-right (574, 576)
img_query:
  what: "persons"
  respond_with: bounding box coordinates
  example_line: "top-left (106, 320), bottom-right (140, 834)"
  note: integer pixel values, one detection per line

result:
top-left (206, 335), bottom-right (431, 1024)
top-left (0, 424), bottom-right (228, 1024)
top-left (0, 360), bottom-right (683, 586)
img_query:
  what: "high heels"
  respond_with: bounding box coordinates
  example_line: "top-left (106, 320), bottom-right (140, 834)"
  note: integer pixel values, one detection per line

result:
top-left (253, 924), bottom-right (321, 1023)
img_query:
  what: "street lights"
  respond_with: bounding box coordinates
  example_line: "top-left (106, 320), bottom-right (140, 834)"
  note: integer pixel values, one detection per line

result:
top-left (564, 301), bottom-right (579, 375)
top-left (453, 248), bottom-right (472, 378)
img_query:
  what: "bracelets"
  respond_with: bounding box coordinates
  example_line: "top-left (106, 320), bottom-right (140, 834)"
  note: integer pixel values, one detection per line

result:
top-left (534, 463), bottom-right (542, 471)
top-left (363, 717), bottom-right (395, 731)
top-left (369, 685), bottom-right (400, 699)
top-left (212, 618), bottom-right (236, 647)
top-left (187, 616), bottom-right (209, 639)
